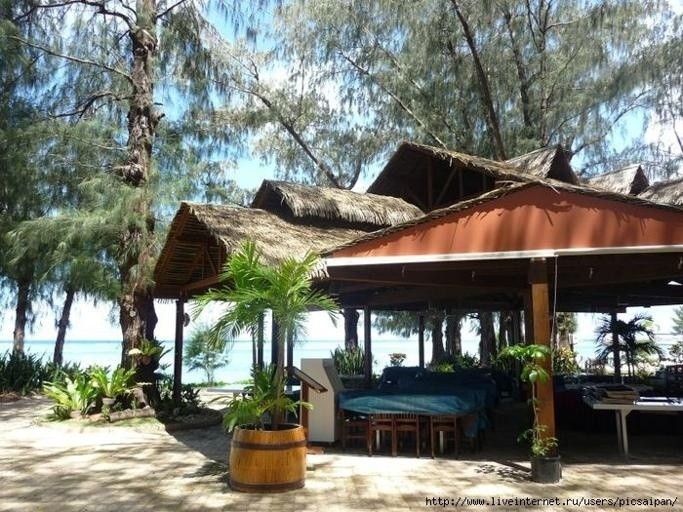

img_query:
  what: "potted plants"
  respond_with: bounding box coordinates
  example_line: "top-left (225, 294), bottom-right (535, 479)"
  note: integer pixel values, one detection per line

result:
top-left (91, 364), bottom-right (137, 405)
top-left (498, 342), bottom-right (561, 482)
top-left (187, 236), bottom-right (342, 493)
top-left (43, 378), bottom-right (85, 417)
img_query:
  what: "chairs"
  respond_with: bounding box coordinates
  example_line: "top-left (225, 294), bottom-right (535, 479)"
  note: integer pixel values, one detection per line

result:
top-left (339, 403), bottom-right (489, 459)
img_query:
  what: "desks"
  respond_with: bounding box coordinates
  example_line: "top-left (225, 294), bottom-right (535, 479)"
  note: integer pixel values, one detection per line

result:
top-left (582, 396), bottom-right (683, 455)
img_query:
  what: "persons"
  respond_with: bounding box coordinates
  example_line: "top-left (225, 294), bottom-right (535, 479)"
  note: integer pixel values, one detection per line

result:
top-left (133, 354), bottom-right (161, 406)
